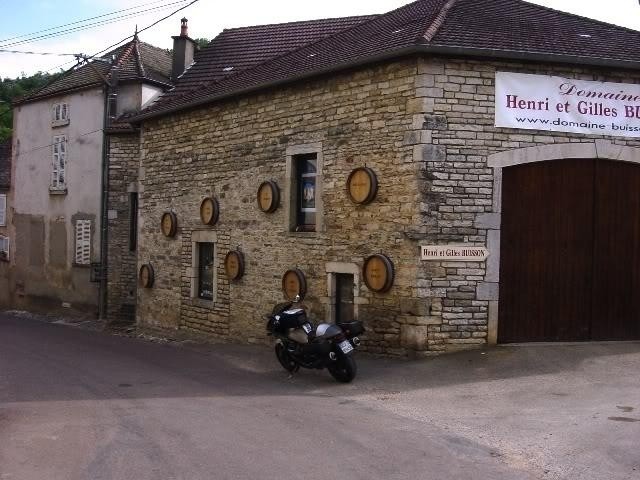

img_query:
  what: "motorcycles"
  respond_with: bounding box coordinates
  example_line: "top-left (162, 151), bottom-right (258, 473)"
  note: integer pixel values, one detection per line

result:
top-left (266, 295), bottom-right (365, 382)
